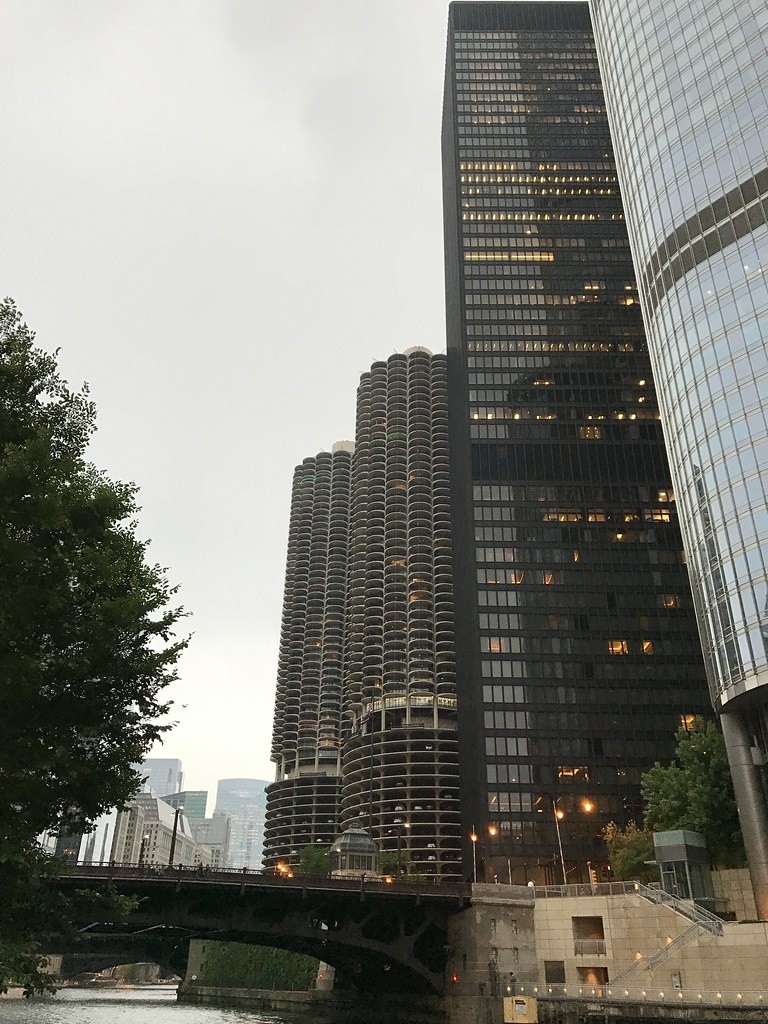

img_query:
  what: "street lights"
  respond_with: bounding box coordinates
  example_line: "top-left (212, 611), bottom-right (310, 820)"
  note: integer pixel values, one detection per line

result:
top-left (552, 801), bottom-right (568, 886)
top-left (137, 834), bottom-right (151, 865)
top-left (167, 806), bottom-right (185, 866)
top-left (470, 824), bottom-right (478, 883)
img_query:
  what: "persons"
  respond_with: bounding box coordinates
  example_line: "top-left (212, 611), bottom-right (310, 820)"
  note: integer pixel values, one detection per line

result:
top-left (503, 972), bottom-right (516, 996)
top-left (197, 860), bottom-right (213, 880)
top-left (364, 873), bottom-right (368, 883)
top-left (528, 879), bottom-right (535, 887)
